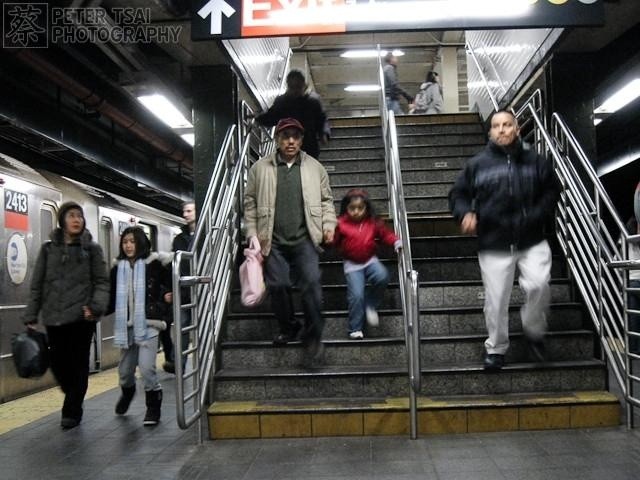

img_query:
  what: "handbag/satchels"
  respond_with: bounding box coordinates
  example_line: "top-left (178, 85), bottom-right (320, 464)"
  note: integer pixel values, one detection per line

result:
top-left (239, 236), bottom-right (267, 308)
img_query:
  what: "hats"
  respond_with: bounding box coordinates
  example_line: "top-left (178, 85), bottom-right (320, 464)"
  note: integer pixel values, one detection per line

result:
top-left (275, 118), bottom-right (305, 136)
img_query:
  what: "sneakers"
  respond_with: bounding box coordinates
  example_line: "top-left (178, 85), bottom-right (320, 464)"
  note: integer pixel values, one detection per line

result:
top-left (366, 306), bottom-right (380, 326)
top-left (350, 331), bottom-right (362, 337)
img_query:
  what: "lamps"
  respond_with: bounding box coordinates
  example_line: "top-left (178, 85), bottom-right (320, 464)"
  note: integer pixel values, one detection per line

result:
top-left (122, 73), bottom-right (194, 148)
top-left (593, 66), bottom-right (639, 127)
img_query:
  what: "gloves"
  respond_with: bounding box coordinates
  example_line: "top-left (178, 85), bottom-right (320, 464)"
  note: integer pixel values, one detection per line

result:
top-left (404, 90), bottom-right (414, 103)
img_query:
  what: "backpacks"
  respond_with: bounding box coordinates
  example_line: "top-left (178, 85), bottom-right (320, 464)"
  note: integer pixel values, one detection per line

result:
top-left (413, 81), bottom-right (434, 112)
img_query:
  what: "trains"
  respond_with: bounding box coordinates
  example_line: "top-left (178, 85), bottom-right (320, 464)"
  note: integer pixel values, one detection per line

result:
top-left (0, 151), bottom-right (195, 406)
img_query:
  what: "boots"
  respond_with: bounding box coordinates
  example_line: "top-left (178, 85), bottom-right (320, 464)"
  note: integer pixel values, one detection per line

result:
top-left (143, 387), bottom-right (163, 426)
top-left (115, 381), bottom-right (138, 415)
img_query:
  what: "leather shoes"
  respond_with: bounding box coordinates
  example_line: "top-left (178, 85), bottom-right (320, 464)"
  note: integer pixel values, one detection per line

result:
top-left (304, 339), bottom-right (324, 360)
top-left (273, 321), bottom-right (297, 345)
top-left (526, 333), bottom-right (555, 362)
top-left (60, 416), bottom-right (82, 427)
top-left (483, 354), bottom-right (504, 373)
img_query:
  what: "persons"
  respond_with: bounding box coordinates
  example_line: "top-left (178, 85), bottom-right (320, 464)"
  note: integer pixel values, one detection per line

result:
top-left (84, 228), bottom-right (168, 428)
top-left (162, 199), bottom-right (199, 376)
top-left (447, 111), bottom-right (563, 369)
top-left (420, 71), bottom-right (445, 113)
top-left (326, 188), bottom-right (403, 339)
top-left (22, 201), bottom-right (110, 431)
top-left (254, 69), bottom-right (330, 157)
top-left (381, 52), bottom-right (415, 115)
top-left (243, 119), bottom-right (338, 364)
